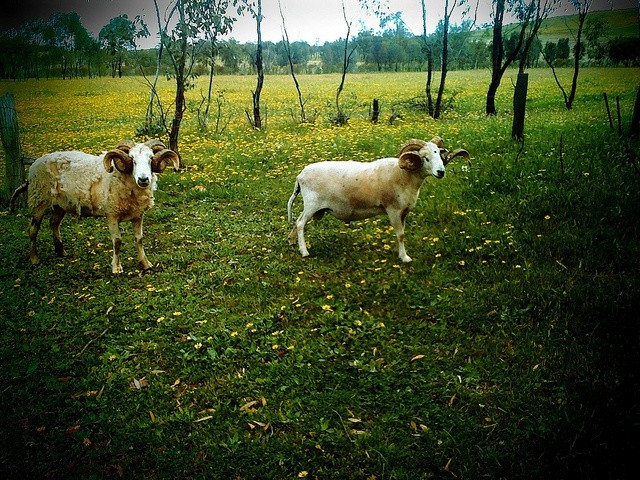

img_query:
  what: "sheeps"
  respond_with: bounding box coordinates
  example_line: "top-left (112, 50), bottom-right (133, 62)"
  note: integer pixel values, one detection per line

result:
top-left (8, 139), bottom-right (180, 278)
top-left (287, 135), bottom-right (472, 267)
